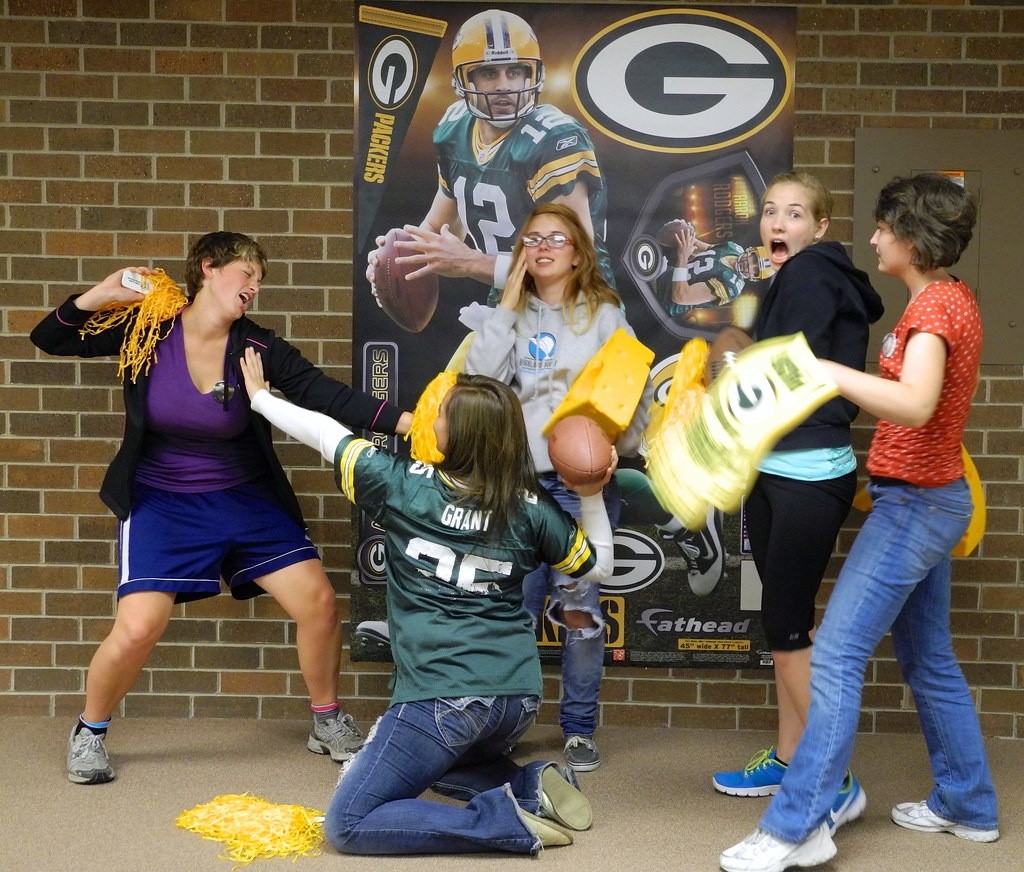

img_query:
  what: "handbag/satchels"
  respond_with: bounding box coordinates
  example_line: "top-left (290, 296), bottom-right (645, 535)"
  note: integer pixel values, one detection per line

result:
top-left (853, 442), bottom-right (986, 559)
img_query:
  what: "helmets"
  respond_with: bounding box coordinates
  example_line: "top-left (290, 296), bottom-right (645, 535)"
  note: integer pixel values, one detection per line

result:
top-left (452, 9), bottom-right (546, 130)
top-left (735, 246), bottom-right (777, 282)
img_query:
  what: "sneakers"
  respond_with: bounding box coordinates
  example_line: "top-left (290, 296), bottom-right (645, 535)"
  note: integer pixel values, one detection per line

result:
top-left (67, 723), bottom-right (116, 785)
top-left (562, 736), bottom-right (600, 771)
top-left (357, 618), bottom-right (392, 651)
top-left (720, 821), bottom-right (836, 872)
top-left (713, 745), bottom-right (787, 796)
top-left (826, 769), bottom-right (866, 837)
top-left (663, 503), bottom-right (731, 597)
top-left (307, 711), bottom-right (365, 761)
top-left (892, 800), bottom-right (999, 843)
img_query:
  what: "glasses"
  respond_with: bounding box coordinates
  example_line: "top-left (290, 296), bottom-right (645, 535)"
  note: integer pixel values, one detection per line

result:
top-left (522, 233), bottom-right (578, 249)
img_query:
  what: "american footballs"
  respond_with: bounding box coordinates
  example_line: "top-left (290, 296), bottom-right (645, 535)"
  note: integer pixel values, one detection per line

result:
top-left (373, 228), bottom-right (438, 333)
top-left (546, 414), bottom-right (615, 486)
top-left (655, 221), bottom-right (695, 250)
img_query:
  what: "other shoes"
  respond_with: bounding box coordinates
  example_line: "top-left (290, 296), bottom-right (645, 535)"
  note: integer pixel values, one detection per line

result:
top-left (517, 807), bottom-right (574, 847)
top-left (542, 766), bottom-right (592, 829)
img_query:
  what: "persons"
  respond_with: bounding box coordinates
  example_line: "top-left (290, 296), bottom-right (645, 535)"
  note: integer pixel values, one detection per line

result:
top-left (30, 232), bottom-right (413, 784)
top-left (700, 171), bottom-right (1004, 870)
top-left (238, 343), bottom-right (619, 859)
top-left (458, 202), bottom-right (657, 771)
top-left (367, 2), bottom-right (757, 597)
